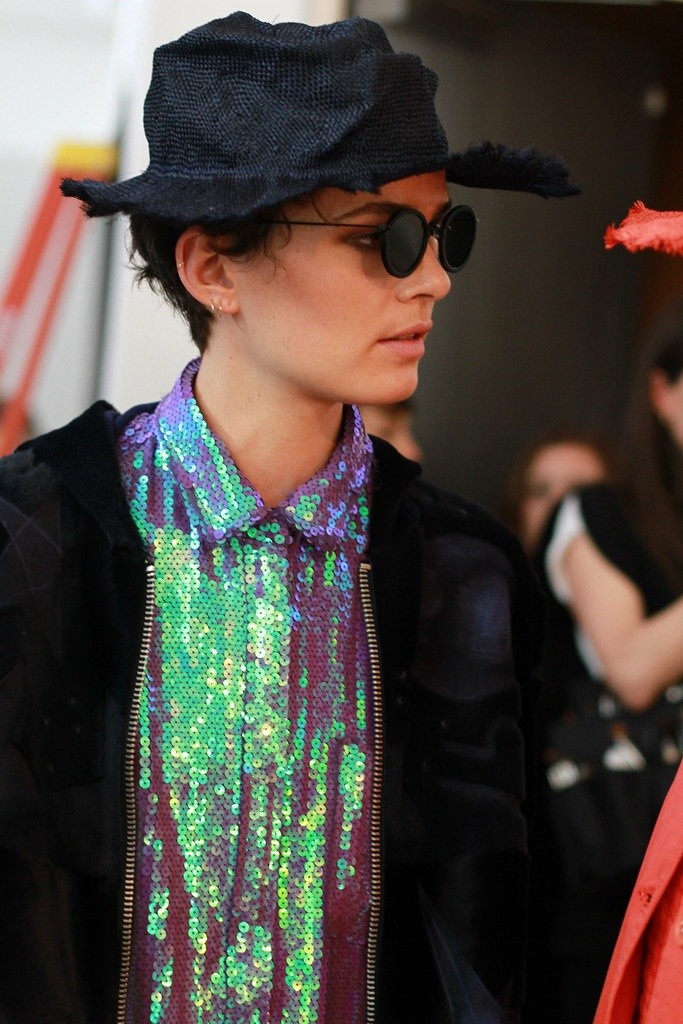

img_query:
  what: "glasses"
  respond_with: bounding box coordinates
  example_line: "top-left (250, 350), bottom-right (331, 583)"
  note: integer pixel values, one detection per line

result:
top-left (249, 203), bottom-right (479, 277)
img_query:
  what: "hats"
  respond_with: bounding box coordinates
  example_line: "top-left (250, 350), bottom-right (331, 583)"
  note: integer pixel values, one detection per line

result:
top-left (58, 10), bottom-right (586, 224)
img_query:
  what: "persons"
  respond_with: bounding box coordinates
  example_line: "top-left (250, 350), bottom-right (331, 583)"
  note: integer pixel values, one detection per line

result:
top-left (525, 198), bottom-right (682, 1024)
top-left (0, 13), bottom-right (588, 1024)
top-left (350, 397), bottom-right (424, 468)
